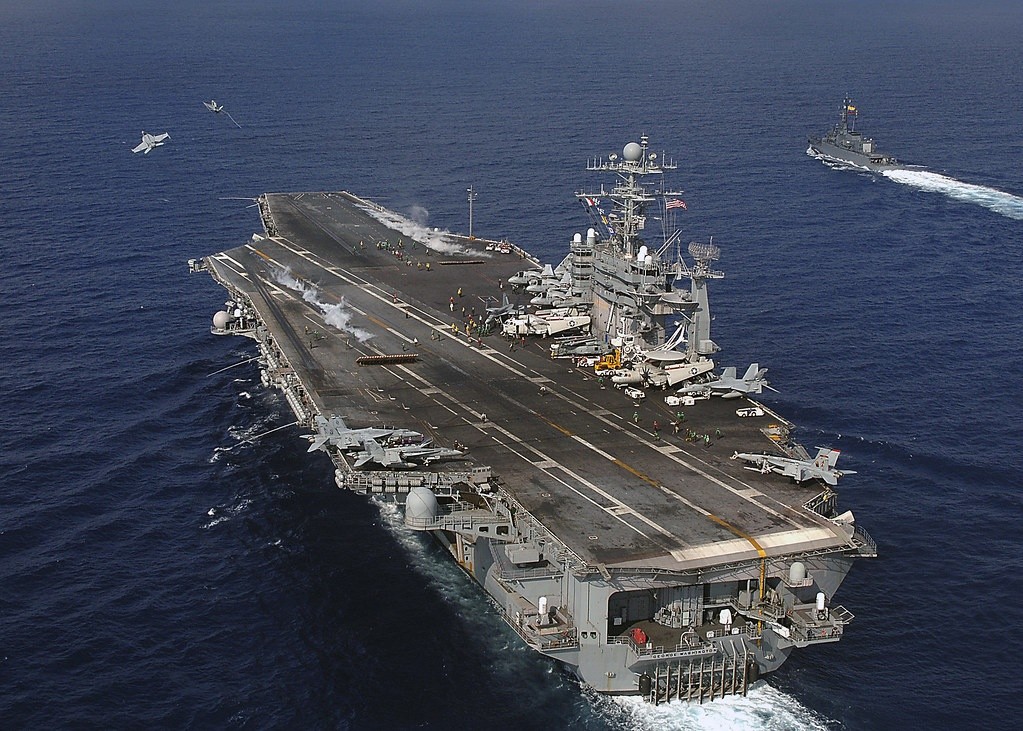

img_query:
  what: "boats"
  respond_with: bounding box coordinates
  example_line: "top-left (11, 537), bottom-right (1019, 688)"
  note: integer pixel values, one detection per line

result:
top-left (804, 91), bottom-right (900, 171)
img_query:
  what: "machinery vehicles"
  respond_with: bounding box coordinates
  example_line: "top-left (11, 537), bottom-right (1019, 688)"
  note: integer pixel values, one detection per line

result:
top-left (595, 349), bottom-right (621, 377)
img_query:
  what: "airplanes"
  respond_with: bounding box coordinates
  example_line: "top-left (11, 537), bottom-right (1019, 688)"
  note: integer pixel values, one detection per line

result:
top-left (484, 293), bottom-right (525, 325)
top-left (676, 363), bottom-right (781, 399)
top-left (201, 96), bottom-right (228, 115)
top-left (134, 130), bottom-right (171, 157)
top-left (730, 446), bottom-right (858, 485)
top-left (524, 263), bottom-right (573, 296)
top-left (346, 435), bottom-right (464, 467)
top-left (611, 363), bottom-right (717, 389)
top-left (299, 414), bottom-right (422, 453)
top-left (506, 253), bottom-right (574, 285)
top-left (530, 283), bottom-right (574, 309)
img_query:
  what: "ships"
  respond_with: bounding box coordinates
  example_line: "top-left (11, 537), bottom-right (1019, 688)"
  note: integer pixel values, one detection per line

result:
top-left (198, 130), bottom-right (877, 706)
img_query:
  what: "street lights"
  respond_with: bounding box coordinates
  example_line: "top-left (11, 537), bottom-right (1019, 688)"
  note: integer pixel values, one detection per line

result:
top-left (467, 184), bottom-right (478, 236)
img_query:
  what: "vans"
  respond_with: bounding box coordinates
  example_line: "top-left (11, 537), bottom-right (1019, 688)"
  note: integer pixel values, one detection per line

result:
top-left (625, 387), bottom-right (646, 399)
top-left (680, 396), bottom-right (695, 406)
top-left (735, 407), bottom-right (764, 418)
top-left (664, 396), bottom-right (679, 406)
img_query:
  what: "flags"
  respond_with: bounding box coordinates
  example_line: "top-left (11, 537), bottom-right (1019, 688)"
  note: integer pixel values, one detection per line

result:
top-left (587, 197), bottom-right (616, 236)
top-left (666, 198), bottom-right (687, 211)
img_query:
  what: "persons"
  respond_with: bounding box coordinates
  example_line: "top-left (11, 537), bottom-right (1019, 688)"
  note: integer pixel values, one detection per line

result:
top-left (425, 248), bottom-right (429, 255)
top-left (401, 342), bottom-right (405, 351)
top-left (633, 412), bottom-right (638, 422)
top-left (405, 309), bottom-right (408, 318)
top-left (478, 338), bottom-right (482, 348)
top-left (540, 385), bottom-right (546, 395)
top-left (598, 376), bottom-right (603, 384)
top-left (411, 241), bottom-right (416, 250)
top-left (417, 261), bottom-right (430, 271)
top-left (305, 326), bottom-right (318, 350)
top-left (715, 428), bottom-right (721, 439)
top-left (674, 411), bottom-right (710, 447)
top-left (449, 297), bottom-right (487, 343)
top-left (498, 278), bottom-right (526, 295)
top-left (377, 240), bottom-right (409, 262)
top-left (458, 288), bottom-right (462, 298)
top-left (346, 332), bottom-right (355, 350)
top-left (480, 412), bottom-right (487, 421)
top-left (360, 241), bottom-right (363, 249)
top-left (414, 337), bottom-right (418, 347)
top-left (654, 431), bottom-right (658, 441)
top-left (431, 330), bottom-right (441, 341)
top-left (508, 342), bottom-right (515, 352)
top-left (653, 419), bottom-right (658, 430)
top-left (452, 440), bottom-right (465, 452)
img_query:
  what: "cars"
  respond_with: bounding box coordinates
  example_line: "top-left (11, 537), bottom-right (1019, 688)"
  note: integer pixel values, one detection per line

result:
top-left (496, 243), bottom-right (510, 254)
top-left (486, 242), bottom-right (494, 251)
